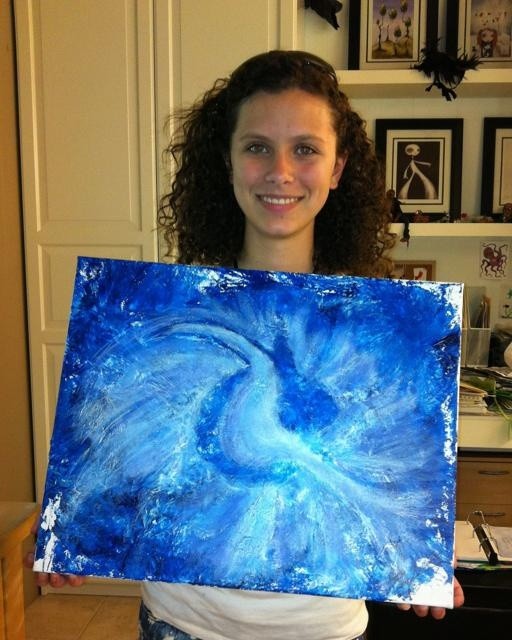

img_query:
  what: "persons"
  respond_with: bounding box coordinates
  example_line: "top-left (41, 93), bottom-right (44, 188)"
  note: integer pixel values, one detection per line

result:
top-left (25, 49), bottom-right (468, 639)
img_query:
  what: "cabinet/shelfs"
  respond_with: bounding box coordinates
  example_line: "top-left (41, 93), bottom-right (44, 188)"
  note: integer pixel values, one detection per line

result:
top-left (456, 457), bottom-right (512, 527)
top-left (14, 0), bottom-right (298, 598)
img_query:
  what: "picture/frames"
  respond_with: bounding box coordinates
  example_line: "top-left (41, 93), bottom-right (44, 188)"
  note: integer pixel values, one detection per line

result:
top-left (374, 116), bottom-right (511, 223)
top-left (347, 0), bottom-right (512, 69)
